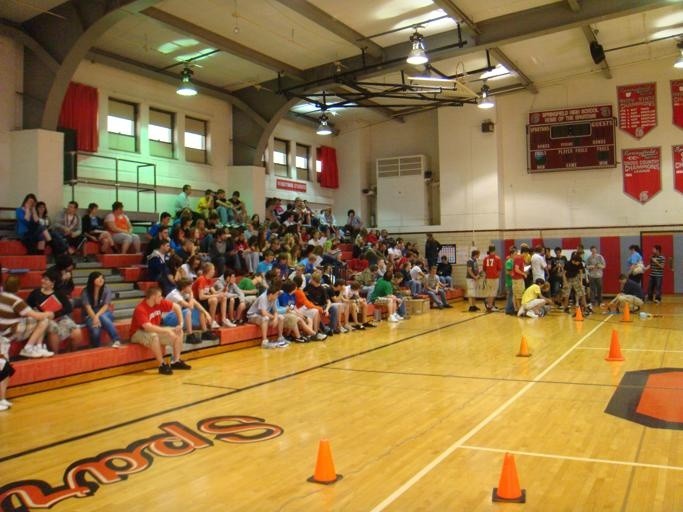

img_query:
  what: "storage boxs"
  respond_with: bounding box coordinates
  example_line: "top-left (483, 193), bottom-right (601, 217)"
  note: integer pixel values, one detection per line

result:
top-left (403, 297), bottom-right (431, 315)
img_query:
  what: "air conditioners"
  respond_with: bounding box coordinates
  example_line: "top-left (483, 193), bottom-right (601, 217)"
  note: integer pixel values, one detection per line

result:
top-left (375, 154), bottom-right (431, 226)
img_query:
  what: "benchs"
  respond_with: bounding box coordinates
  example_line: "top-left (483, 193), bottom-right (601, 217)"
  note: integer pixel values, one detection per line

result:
top-left (0, 205), bottom-right (466, 398)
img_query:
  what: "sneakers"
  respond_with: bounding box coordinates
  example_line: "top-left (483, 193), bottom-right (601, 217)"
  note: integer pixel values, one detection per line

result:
top-left (20, 344), bottom-right (54, 358)
top-left (212, 321), bottom-right (219, 329)
top-left (526, 311), bottom-right (538, 317)
top-left (517, 307), bottom-right (524, 316)
top-left (185, 334), bottom-right (200, 344)
top-left (469, 305), bottom-right (498, 312)
top-left (172, 358), bottom-right (192, 370)
top-left (200, 331), bottom-right (217, 340)
top-left (223, 319), bottom-right (237, 328)
top-left (309, 323), bottom-right (375, 340)
top-left (261, 335), bottom-right (309, 347)
top-left (159, 360), bottom-right (172, 374)
top-left (112, 342), bottom-right (120, 348)
top-left (389, 313), bottom-right (403, 322)
top-left (0, 399), bottom-right (12, 411)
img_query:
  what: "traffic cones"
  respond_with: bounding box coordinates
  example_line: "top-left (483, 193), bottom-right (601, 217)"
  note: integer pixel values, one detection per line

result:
top-left (571, 305), bottom-right (586, 323)
top-left (619, 300), bottom-right (634, 323)
top-left (516, 333), bottom-right (532, 358)
top-left (309, 435), bottom-right (344, 487)
top-left (491, 451), bottom-right (529, 505)
top-left (603, 329), bottom-right (625, 362)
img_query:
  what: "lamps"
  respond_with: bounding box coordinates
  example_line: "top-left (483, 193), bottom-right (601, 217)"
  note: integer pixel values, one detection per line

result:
top-left (175, 60), bottom-right (200, 97)
top-left (314, 88), bottom-right (334, 138)
top-left (406, 25), bottom-right (427, 66)
top-left (477, 79), bottom-right (496, 110)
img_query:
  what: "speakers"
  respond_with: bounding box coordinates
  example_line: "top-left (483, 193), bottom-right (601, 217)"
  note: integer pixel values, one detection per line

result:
top-left (58, 128), bottom-right (77, 185)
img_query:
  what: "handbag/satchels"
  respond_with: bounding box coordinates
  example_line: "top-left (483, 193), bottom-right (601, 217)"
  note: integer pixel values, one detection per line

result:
top-left (632, 263), bottom-right (643, 275)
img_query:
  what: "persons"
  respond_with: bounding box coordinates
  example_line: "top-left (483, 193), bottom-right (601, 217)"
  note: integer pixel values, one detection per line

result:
top-left (464, 240), bottom-right (666, 318)
top-left (17, 193), bottom-right (141, 260)
top-left (0, 256), bottom-right (121, 410)
top-left (129, 286), bottom-right (191, 375)
top-left (145, 184), bottom-right (455, 349)
